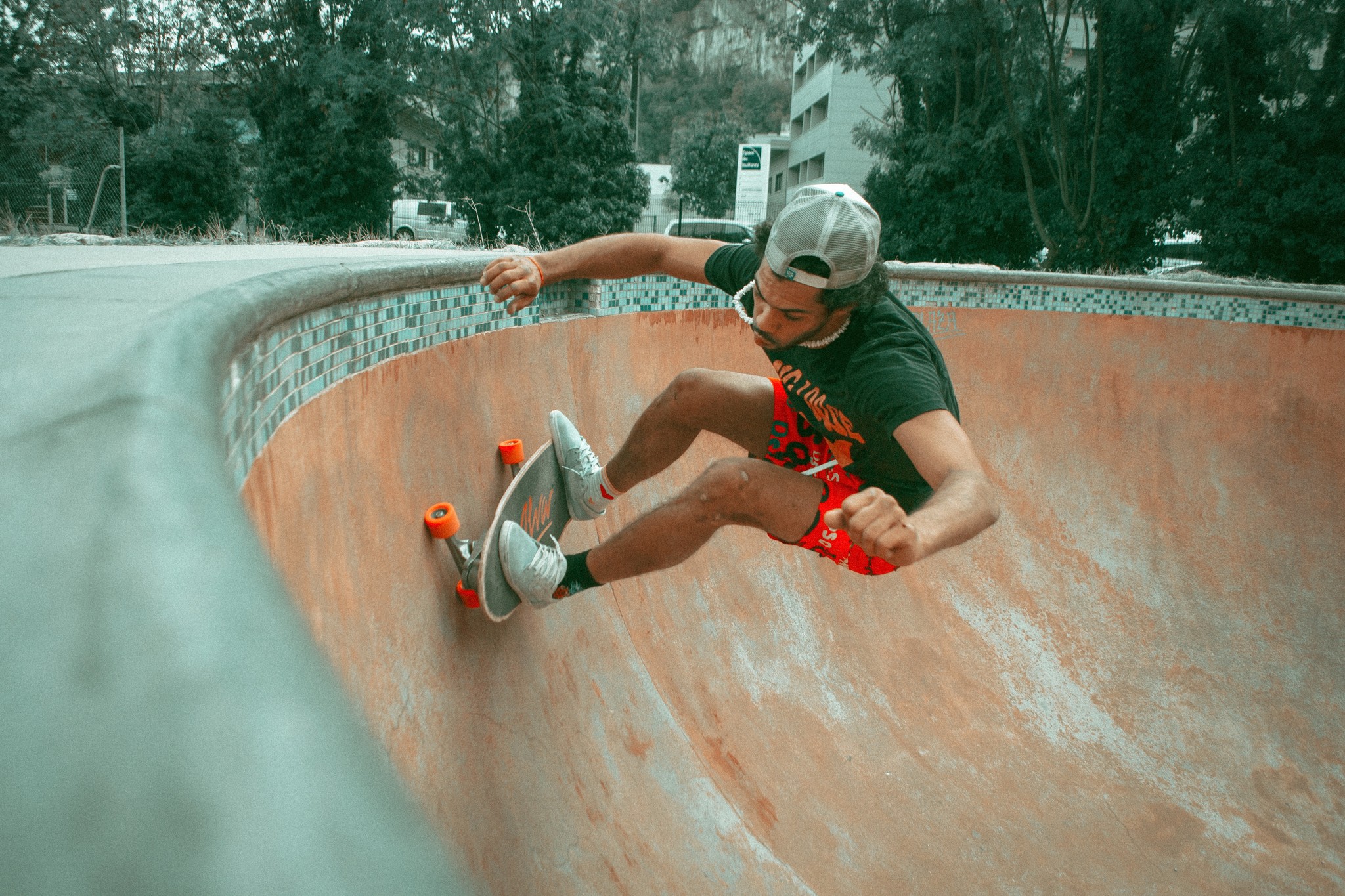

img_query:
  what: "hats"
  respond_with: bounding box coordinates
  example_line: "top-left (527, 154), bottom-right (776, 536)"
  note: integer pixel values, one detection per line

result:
top-left (765, 181), bottom-right (881, 291)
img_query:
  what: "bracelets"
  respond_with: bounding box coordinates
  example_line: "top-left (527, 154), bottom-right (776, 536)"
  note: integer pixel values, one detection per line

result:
top-left (525, 256), bottom-right (544, 287)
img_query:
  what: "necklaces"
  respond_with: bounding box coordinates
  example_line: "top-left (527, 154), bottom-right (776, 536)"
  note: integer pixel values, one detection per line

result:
top-left (731, 278), bottom-right (850, 347)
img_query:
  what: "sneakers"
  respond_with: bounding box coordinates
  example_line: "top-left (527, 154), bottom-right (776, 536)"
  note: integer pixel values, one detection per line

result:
top-left (548, 409), bottom-right (607, 521)
top-left (499, 520), bottom-right (565, 612)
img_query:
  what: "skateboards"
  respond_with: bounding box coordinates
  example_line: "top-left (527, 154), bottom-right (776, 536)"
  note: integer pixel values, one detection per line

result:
top-left (424, 439), bottom-right (572, 623)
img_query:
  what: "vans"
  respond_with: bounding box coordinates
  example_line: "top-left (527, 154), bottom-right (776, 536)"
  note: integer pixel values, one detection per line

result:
top-left (664, 218), bottom-right (758, 246)
top-left (386, 198), bottom-right (506, 249)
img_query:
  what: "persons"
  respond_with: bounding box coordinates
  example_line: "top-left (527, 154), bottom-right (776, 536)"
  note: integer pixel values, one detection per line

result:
top-left (481, 181), bottom-right (1000, 613)
